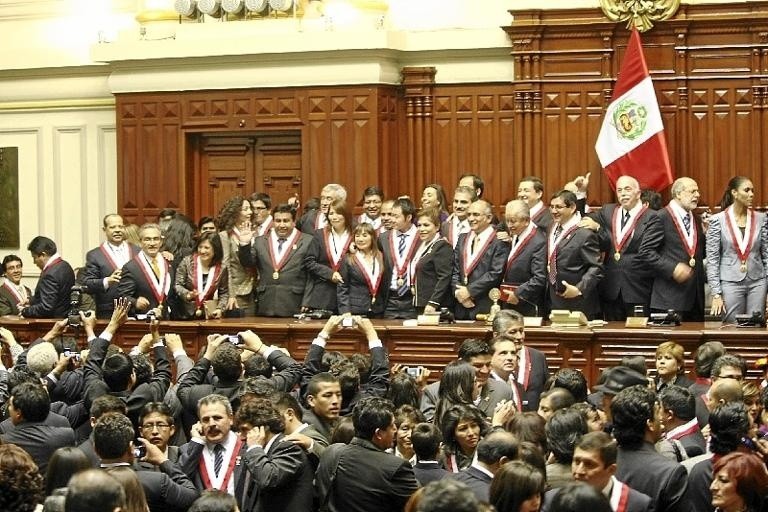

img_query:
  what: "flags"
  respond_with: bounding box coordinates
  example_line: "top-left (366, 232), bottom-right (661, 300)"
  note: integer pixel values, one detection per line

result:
top-left (595, 20), bottom-right (673, 200)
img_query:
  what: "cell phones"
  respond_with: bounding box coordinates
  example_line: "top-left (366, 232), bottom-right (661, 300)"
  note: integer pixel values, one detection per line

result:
top-left (259, 424), bottom-right (270, 433)
top-left (195, 421), bottom-right (204, 437)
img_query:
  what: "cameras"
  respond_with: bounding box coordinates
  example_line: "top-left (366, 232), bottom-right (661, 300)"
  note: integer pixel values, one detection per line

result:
top-left (135, 309), bottom-right (158, 323)
top-left (61, 348), bottom-right (81, 372)
top-left (63, 284), bottom-right (85, 332)
top-left (223, 336), bottom-right (241, 346)
top-left (405, 367), bottom-right (424, 379)
top-left (132, 446), bottom-right (145, 458)
top-left (340, 314), bottom-right (358, 330)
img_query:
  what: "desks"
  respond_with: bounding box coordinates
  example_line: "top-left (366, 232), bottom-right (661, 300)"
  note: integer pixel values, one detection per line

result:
top-left (0, 317), bottom-right (768, 391)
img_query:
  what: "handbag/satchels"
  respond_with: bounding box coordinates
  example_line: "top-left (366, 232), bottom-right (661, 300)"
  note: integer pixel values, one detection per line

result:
top-left (293, 310), bottom-right (333, 319)
top-left (555, 270), bottom-right (582, 291)
top-left (203, 299), bottom-right (219, 321)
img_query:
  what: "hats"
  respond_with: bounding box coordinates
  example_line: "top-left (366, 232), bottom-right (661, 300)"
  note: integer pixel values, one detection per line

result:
top-left (592, 366), bottom-right (649, 395)
top-left (754, 356), bottom-right (767, 368)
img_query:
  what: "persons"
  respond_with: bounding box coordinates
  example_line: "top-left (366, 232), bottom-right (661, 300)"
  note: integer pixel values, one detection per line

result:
top-left (1, 176), bottom-right (768, 317)
top-left (3, 297), bottom-right (767, 512)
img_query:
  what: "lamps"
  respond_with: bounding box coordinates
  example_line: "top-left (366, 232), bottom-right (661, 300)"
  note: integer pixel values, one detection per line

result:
top-left (245, 0), bottom-right (273, 21)
top-left (270, 0), bottom-right (298, 19)
top-left (197, 0), bottom-right (225, 22)
top-left (222, 0), bottom-right (249, 21)
top-left (175, 0), bottom-right (202, 24)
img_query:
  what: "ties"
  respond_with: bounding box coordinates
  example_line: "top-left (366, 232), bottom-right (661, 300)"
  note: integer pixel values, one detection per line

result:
top-left (683, 213), bottom-right (690, 237)
top-left (554, 226), bottom-right (563, 243)
top-left (514, 237), bottom-right (519, 247)
top-left (397, 234), bottom-right (409, 298)
top-left (277, 238), bottom-right (288, 254)
top-left (369, 221), bottom-right (376, 228)
top-left (513, 356), bottom-right (519, 378)
top-left (19, 287), bottom-right (25, 299)
top-left (473, 236), bottom-right (480, 254)
top-left (456, 223), bottom-right (464, 238)
top-left (152, 258), bottom-right (160, 281)
top-left (623, 212), bottom-right (630, 228)
top-left (213, 443), bottom-right (223, 479)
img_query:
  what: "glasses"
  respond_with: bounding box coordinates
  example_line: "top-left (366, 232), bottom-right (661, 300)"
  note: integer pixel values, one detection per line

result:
top-left (681, 189), bottom-right (701, 196)
top-left (141, 422), bottom-right (171, 431)
top-left (759, 406), bottom-right (765, 410)
top-left (141, 237), bottom-right (161, 242)
top-left (201, 226), bottom-right (216, 231)
top-left (363, 200), bottom-right (382, 205)
top-left (252, 207), bottom-right (267, 213)
top-left (711, 375), bottom-right (743, 381)
top-left (549, 204), bottom-right (571, 212)
